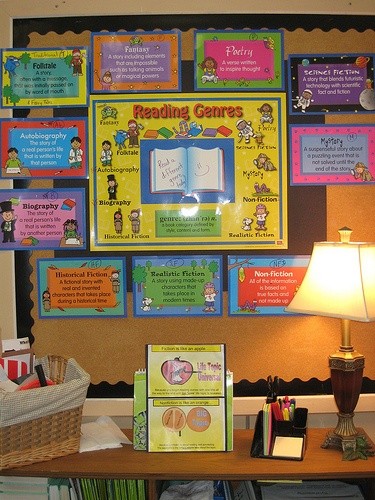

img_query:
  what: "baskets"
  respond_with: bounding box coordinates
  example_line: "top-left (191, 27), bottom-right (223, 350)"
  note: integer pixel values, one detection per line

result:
top-left (0, 356), bottom-right (90, 469)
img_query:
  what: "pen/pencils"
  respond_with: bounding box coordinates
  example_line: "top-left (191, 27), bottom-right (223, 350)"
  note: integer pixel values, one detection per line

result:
top-left (264, 373), bottom-right (296, 421)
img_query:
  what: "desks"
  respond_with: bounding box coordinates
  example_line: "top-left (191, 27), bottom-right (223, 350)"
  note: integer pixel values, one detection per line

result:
top-left (0, 422), bottom-right (375, 500)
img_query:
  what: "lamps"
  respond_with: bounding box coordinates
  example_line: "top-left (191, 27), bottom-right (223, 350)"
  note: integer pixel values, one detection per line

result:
top-left (285, 225), bottom-right (375, 452)
